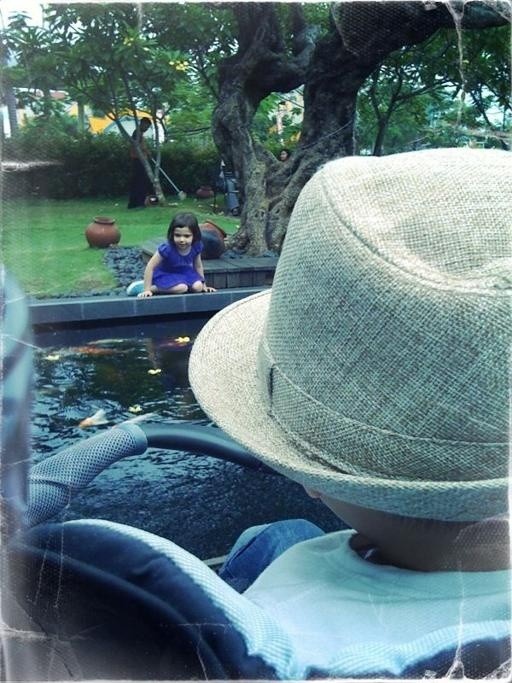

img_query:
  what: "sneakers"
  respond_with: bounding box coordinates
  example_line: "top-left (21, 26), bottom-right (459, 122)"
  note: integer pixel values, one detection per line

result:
top-left (126, 279), bottom-right (144, 296)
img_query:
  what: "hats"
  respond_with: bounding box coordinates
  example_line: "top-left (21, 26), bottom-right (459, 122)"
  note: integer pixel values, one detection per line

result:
top-left (187, 148), bottom-right (508, 525)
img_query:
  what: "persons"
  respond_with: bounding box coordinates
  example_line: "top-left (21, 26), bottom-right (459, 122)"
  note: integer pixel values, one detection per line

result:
top-left (128, 117), bottom-right (152, 209)
top-left (126, 212), bottom-right (217, 298)
top-left (277, 149), bottom-right (290, 162)
top-left (189, 147), bottom-right (512, 682)
